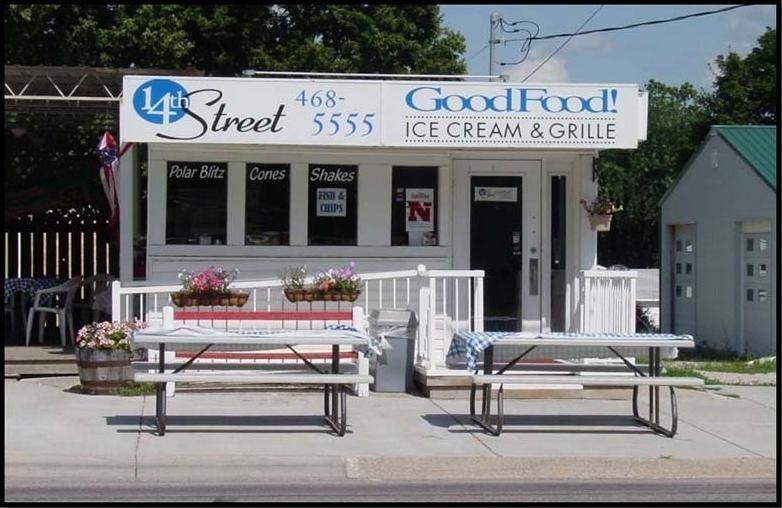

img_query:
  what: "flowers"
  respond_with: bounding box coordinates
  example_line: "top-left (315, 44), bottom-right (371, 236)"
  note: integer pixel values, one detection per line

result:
top-left (177, 265), bottom-right (240, 296)
top-left (579, 191), bottom-right (624, 216)
top-left (276, 262), bottom-right (368, 295)
top-left (75, 316), bottom-right (149, 349)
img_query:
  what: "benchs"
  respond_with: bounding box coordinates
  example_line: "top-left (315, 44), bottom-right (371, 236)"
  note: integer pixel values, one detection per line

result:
top-left (132, 360), bottom-right (376, 436)
top-left (454, 363), bottom-right (705, 438)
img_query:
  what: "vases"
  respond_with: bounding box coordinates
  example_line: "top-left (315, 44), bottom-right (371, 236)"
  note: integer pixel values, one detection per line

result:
top-left (588, 216), bottom-right (613, 231)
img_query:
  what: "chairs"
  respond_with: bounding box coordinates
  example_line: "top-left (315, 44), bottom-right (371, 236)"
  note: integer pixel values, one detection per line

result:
top-left (24, 273), bottom-right (120, 348)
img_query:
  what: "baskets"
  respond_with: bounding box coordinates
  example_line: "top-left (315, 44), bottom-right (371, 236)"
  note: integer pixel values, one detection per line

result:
top-left (170, 292), bottom-right (250, 307)
top-left (285, 289), bottom-right (361, 301)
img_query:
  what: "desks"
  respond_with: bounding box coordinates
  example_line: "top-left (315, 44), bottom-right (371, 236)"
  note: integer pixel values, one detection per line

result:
top-left (129, 332), bottom-right (370, 436)
top-left (453, 333), bottom-right (698, 439)
